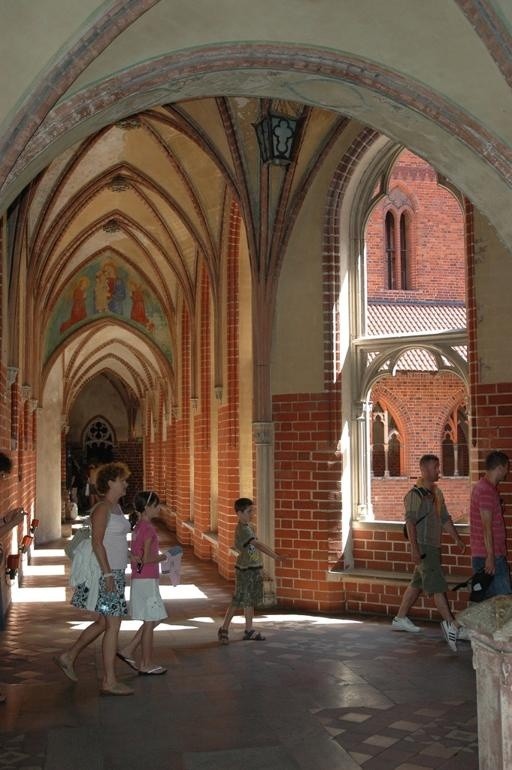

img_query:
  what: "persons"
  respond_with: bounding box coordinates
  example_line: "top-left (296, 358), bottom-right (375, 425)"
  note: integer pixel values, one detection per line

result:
top-left (217, 497), bottom-right (283, 644)
top-left (389, 454), bottom-right (467, 636)
top-left (54, 461), bottom-right (135, 696)
top-left (440, 450), bottom-right (510, 653)
top-left (84, 456), bottom-right (100, 505)
top-left (117, 492), bottom-right (183, 676)
top-left (66, 455), bottom-right (84, 515)
top-left (1, 453), bottom-right (27, 539)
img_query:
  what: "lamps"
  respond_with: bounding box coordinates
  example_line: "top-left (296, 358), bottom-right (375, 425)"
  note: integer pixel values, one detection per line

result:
top-left (250, 108), bottom-right (299, 165)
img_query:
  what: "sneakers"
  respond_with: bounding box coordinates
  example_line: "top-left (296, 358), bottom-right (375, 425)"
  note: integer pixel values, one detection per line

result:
top-left (392, 614), bottom-right (422, 633)
top-left (441, 620), bottom-right (458, 652)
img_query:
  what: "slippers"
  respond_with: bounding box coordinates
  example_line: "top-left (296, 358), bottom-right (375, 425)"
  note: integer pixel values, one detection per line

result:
top-left (115, 650), bottom-right (139, 670)
top-left (134, 665), bottom-right (165, 676)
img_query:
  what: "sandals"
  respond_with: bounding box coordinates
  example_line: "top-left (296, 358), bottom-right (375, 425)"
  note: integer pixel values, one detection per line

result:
top-left (101, 680), bottom-right (132, 695)
top-left (52, 653), bottom-right (79, 681)
top-left (242, 630), bottom-right (265, 640)
top-left (218, 626), bottom-right (231, 645)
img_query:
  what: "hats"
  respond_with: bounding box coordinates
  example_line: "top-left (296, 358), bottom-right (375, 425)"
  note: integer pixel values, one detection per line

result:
top-left (469, 569), bottom-right (495, 603)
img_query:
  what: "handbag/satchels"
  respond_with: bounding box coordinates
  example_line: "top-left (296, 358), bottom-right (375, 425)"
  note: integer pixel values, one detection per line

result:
top-left (63, 525), bottom-right (90, 559)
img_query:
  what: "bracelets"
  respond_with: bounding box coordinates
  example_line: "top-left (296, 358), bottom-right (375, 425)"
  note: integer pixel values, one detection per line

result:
top-left (102, 570), bottom-right (116, 579)
top-left (3, 516), bottom-right (9, 524)
